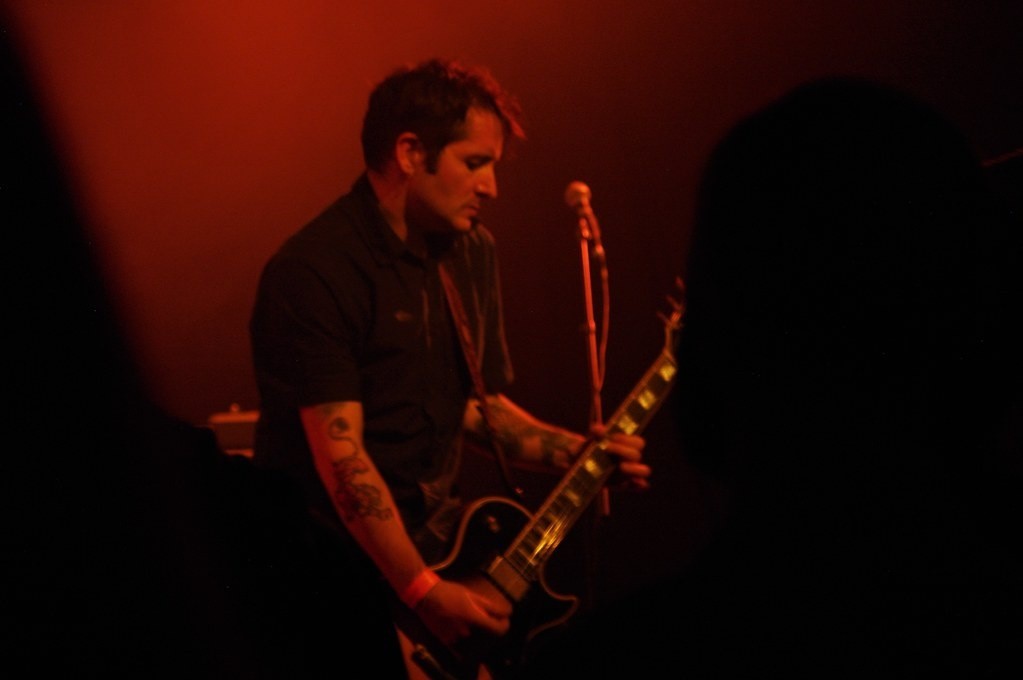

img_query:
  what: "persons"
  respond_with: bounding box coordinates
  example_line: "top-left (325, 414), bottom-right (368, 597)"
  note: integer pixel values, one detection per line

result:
top-left (251, 60), bottom-right (651, 663)
top-left (510, 76), bottom-right (1022, 679)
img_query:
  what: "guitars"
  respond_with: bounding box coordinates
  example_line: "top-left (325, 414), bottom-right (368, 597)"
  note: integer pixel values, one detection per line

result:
top-left (389, 273), bottom-right (690, 680)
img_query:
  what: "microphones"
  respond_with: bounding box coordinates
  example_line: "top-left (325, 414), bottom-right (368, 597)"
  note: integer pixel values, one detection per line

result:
top-left (562, 178), bottom-right (600, 243)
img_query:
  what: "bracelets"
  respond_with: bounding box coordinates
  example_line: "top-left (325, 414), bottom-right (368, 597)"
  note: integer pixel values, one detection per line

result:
top-left (402, 569), bottom-right (439, 607)
